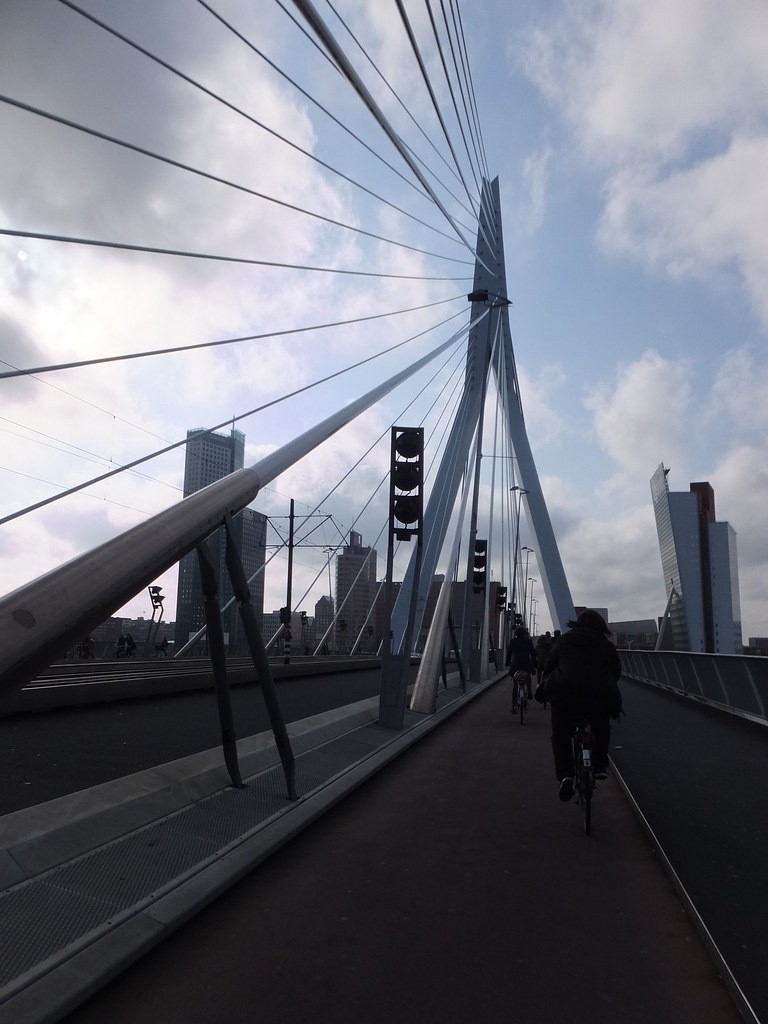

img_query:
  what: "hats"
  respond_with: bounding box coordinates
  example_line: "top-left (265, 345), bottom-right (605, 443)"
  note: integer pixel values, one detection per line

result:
top-left (568, 610), bottom-right (611, 635)
top-left (515, 627), bottom-right (528, 636)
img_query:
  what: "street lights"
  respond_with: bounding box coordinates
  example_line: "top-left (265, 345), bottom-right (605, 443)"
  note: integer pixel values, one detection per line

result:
top-left (521, 545), bottom-right (534, 627)
top-left (528, 575), bottom-right (539, 635)
top-left (510, 487), bottom-right (530, 639)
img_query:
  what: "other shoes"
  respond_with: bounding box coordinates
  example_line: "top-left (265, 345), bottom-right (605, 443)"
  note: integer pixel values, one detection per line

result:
top-left (558, 777), bottom-right (573, 801)
top-left (595, 767), bottom-right (608, 778)
top-left (528, 691), bottom-right (533, 700)
top-left (511, 708), bottom-right (517, 714)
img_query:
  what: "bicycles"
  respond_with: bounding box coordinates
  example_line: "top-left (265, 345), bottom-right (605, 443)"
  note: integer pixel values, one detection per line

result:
top-left (112, 644), bottom-right (135, 659)
top-left (542, 698), bottom-right (605, 837)
top-left (76, 644), bottom-right (88, 660)
top-left (535, 663), bottom-right (551, 711)
top-left (508, 660), bottom-right (537, 725)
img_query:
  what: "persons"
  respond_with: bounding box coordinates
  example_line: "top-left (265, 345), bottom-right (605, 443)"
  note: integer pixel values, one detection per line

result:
top-left (534, 609), bottom-right (623, 801)
top-left (505, 625), bottom-right (563, 714)
top-left (154, 637), bottom-right (169, 657)
top-left (114, 633), bottom-right (137, 658)
top-left (65, 638), bottom-right (94, 659)
